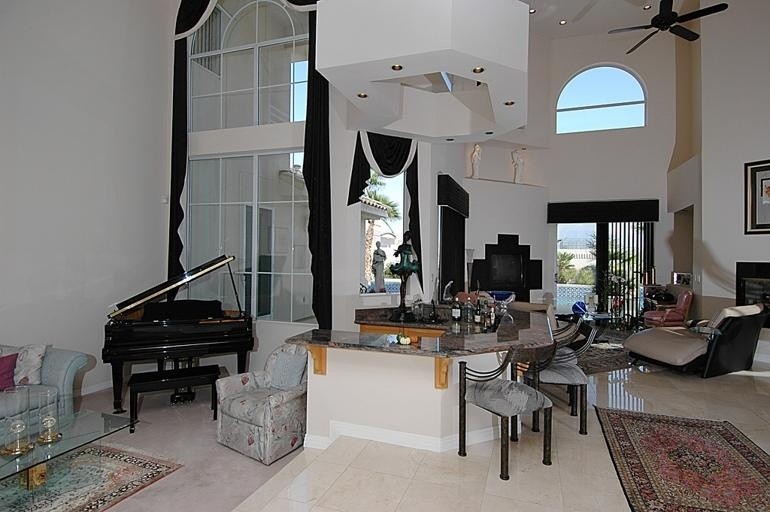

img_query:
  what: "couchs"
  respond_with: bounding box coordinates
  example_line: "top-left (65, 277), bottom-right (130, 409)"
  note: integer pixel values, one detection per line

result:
top-left (0, 344), bottom-right (89, 447)
top-left (622, 303), bottom-right (770, 378)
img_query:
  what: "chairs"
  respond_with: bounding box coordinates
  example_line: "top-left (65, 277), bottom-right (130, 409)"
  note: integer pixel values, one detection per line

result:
top-left (541, 315), bottom-right (584, 406)
top-left (215, 343), bottom-right (308, 466)
top-left (457, 340), bottom-right (559, 481)
top-left (524, 323), bottom-right (597, 434)
top-left (644, 290), bottom-right (694, 328)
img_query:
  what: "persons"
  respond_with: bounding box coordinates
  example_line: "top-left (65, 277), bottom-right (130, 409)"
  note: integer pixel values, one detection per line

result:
top-left (372, 241), bottom-right (386, 292)
top-left (471, 144), bottom-right (481, 179)
top-left (389, 231), bottom-right (421, 275)
top-left (512, 149), bottom-right (524, 182)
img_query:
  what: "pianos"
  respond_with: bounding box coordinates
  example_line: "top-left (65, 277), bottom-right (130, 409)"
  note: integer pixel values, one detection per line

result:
top-left (102, 254), bottom-right (255, 414)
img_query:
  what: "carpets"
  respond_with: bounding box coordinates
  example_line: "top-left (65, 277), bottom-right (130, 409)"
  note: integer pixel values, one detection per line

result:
top-left (575, 342), bottom-right (645, 374)
top-left (594, 406), bottom-right (770, 512)
top-left (0, 443), bottom-right (184, 512)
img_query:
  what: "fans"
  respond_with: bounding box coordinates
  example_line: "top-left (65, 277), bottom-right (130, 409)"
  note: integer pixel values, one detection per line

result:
top-left (608, 0), bottom-right (728, 55)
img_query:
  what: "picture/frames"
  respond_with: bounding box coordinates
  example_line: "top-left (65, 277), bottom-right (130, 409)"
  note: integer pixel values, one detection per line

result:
top-left (743, 159), bottom-right (770, 235)
top-left (674, 272), bottom-right (692, 287)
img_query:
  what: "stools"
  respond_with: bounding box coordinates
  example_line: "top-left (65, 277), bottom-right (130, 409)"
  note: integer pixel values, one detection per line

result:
top-left (128, 364), bottom-right (221, 434)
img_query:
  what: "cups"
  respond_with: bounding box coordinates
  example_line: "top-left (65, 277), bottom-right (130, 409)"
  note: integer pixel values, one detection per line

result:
top-left (4, 385), bottom-right (31, 451)
top-left (38, 387), bottom-right (59, 443)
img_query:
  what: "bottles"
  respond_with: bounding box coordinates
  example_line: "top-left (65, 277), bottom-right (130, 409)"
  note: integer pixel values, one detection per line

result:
top-left (451, 321), bottom-right (460, 337)
top-left (448, 297), bottom-right (507, 329)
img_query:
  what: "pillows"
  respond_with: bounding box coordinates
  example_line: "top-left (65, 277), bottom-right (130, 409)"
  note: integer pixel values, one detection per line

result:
top-left (13, 344), bottom-right (48, 385)
top-left (0, 352), bottom-right (18, 393)
top-left (688, 327), bottom-right (722, 336)
